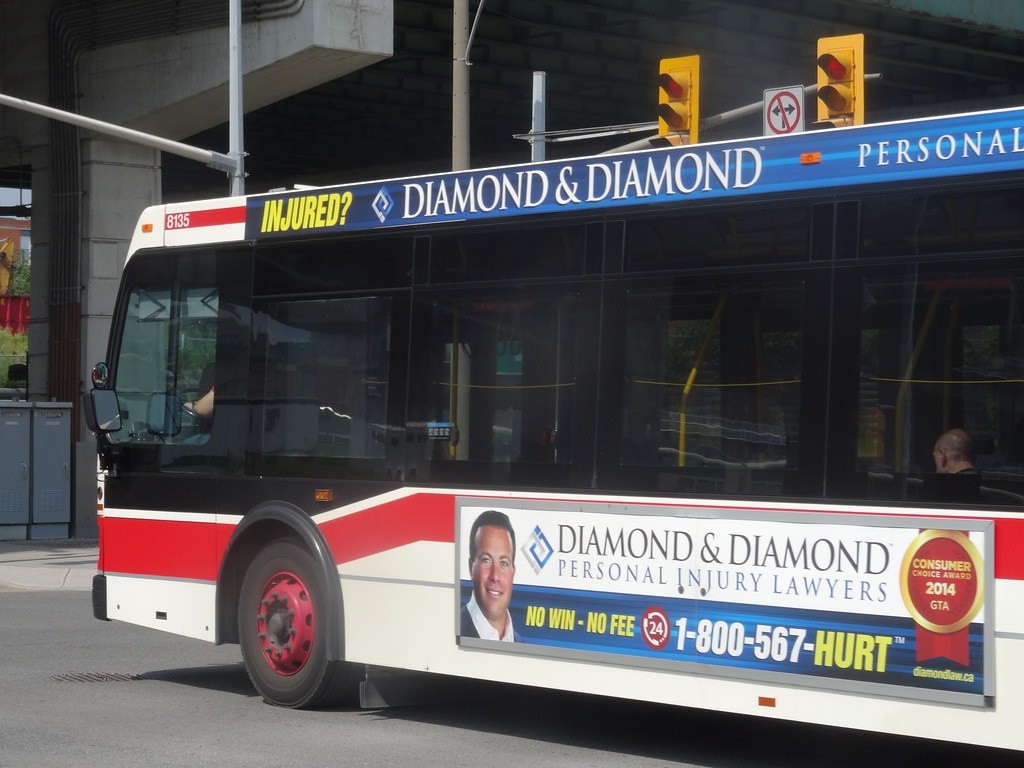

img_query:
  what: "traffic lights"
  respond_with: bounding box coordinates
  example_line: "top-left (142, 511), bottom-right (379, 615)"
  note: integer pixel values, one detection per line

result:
top-left (811, 33), bottom-right (865, 130)
top-left (648, 54), bottom-right (700, 148)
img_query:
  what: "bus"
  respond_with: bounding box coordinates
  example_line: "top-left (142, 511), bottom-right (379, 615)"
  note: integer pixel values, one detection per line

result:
top-left (79, 105), bottom-right (1023, 754)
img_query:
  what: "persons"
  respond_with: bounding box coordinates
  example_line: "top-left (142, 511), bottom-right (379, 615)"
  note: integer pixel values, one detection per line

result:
top-left (921, 426), bottom-right (981, 474)
top-left (180, 381), bottom-right (216, 421)
top-left (460, 509), bottom-right (525, 643)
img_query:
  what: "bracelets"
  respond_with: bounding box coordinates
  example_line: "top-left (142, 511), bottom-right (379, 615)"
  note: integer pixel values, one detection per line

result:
top-left (192, 399), bottom-right (196, 412)
